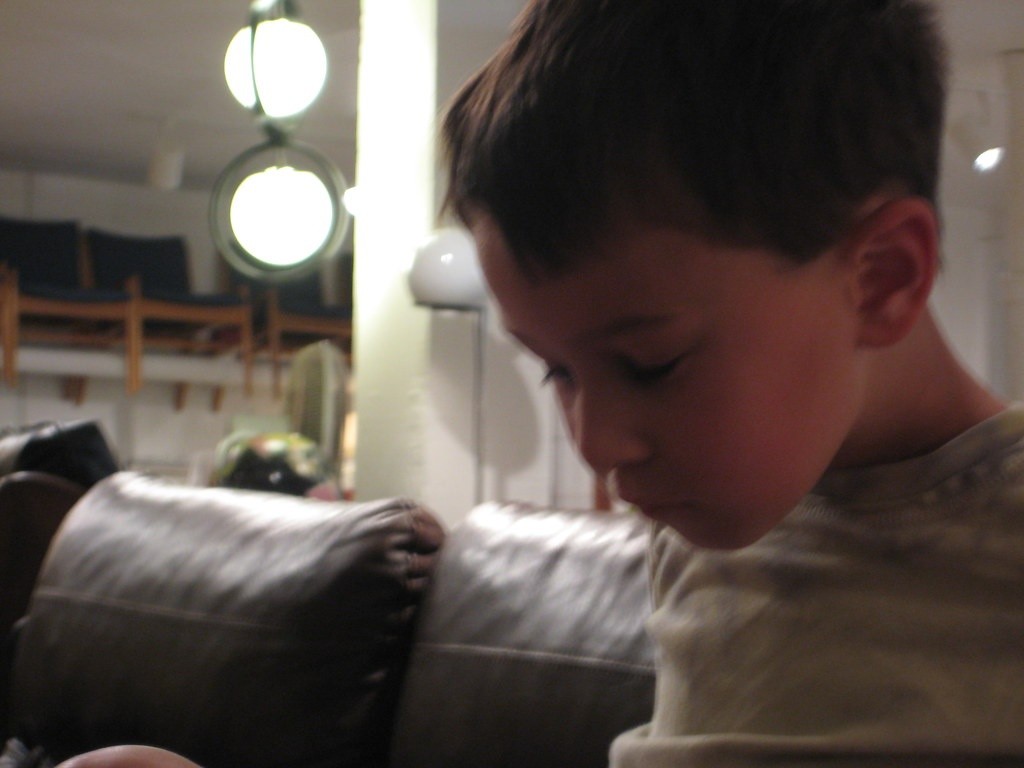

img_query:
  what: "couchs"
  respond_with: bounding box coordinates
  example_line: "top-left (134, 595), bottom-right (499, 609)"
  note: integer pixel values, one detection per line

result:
top-left (0, 419), bottom-right (685, 768)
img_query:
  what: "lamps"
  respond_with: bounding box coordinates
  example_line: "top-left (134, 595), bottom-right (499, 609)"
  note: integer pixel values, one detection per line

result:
top-left (211, 0), bottom-right (348, 283)
top-left (411, 232), bottom-right (490, 505)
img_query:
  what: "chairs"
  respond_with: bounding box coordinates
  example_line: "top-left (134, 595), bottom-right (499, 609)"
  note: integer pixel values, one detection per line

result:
top-left (0, 210), bottom-right (351, 412)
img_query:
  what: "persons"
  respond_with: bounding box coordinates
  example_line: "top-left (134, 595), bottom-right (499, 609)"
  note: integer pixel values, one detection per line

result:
top-left (431, 0), bottom-right (1024, 768)
